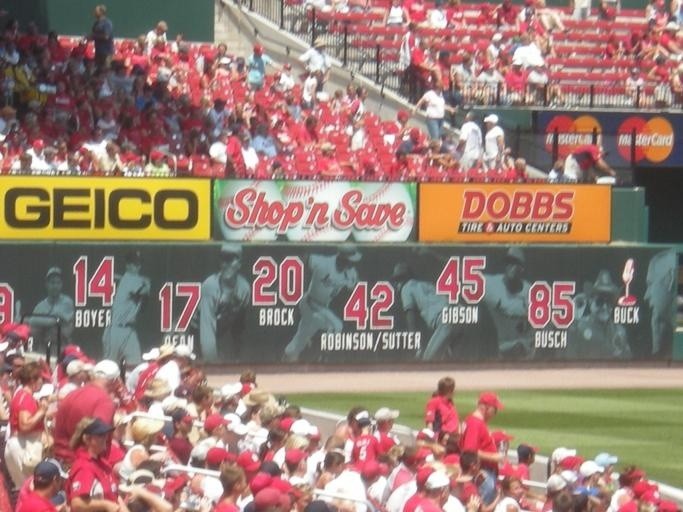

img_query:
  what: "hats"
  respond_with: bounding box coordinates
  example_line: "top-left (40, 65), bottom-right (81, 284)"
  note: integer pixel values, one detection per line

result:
top-left (546, 447), bottom-right (677, 512)
top-left (0, 32), bottom-right (454, 185)
top-left (491, 431), bottom-right (512, 445)
top-left (483, 114), bottom-right (498, 123)
top-left (131, 397), bottom-right (197, 438)
top-left (337, 244), bottom-right (361, 262)
top-left (452, 2), bottom-right (681, 76)
top-left (46, 266), bottom-right (61, 278)
top-left (505, 247), bottom-right (524, 267)
top-left (208, 449), bottom-right (302, 509)
top-left (126, 250), bottom-right (144, 263)
top-left (517, 444), bottom-right (538, 457)
top-left (82, 418), bottom-right (116, 436)
top-left (479, 392), bottom-right (503, 410)
top-left (0, 341), bottom-right (196, 401)
top-left (349, 407), bottom-right (370, 426)
top-left (281, 418), bottom-right (322, 466)
top-left (407, 448), bottom-right (450, 490)
top-left (221, 244), bottom-right (242, 260)
top-left (202, 382), bottom-right (286, 434)
top-left (33, 462), bottom-right (58, 480)
top-left (374, 408), bottom-right (398, 421)
top-left (43, 458), bottom-right (70, 479)
top-left (360, 460), bottom-right (388, 478)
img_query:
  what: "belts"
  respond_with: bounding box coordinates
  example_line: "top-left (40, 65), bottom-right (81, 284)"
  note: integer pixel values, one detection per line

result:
top-left (118, 322), bottom-right (134, 327)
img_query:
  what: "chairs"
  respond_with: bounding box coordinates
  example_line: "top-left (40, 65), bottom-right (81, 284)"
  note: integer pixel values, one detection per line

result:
top-left (285, 0), bottom-right (683, 108)
top-left (1, 32), bottom-right (545, 183)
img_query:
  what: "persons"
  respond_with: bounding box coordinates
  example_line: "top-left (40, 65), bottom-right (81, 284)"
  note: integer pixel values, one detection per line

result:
top-left (1, 1), bottom-right (47, 33)
top-left (477, 246), bottom-right (546, 359)
top-left (391, 261), bottom-right (466, 362)
top-left (22, 265), bottom-right (75, 357)
top-left (0, 319), bottom-right (681, 512)
top-left (83, 5), bottom-right (113, 75)
top-left (102, 249), bottom-right (151, 372)
top-left (186, 242), bottom-right (253, 363)
top-left (281, 242), bottom-right (363, 363)
top-left (640, 247), bottom-right (682, 361)
top-left (564, 267), bottom-right (634, 359)
top-left (1, 16), bottom-right (616, 186)
top-left (283, 0), bottom-right (682, 108)
